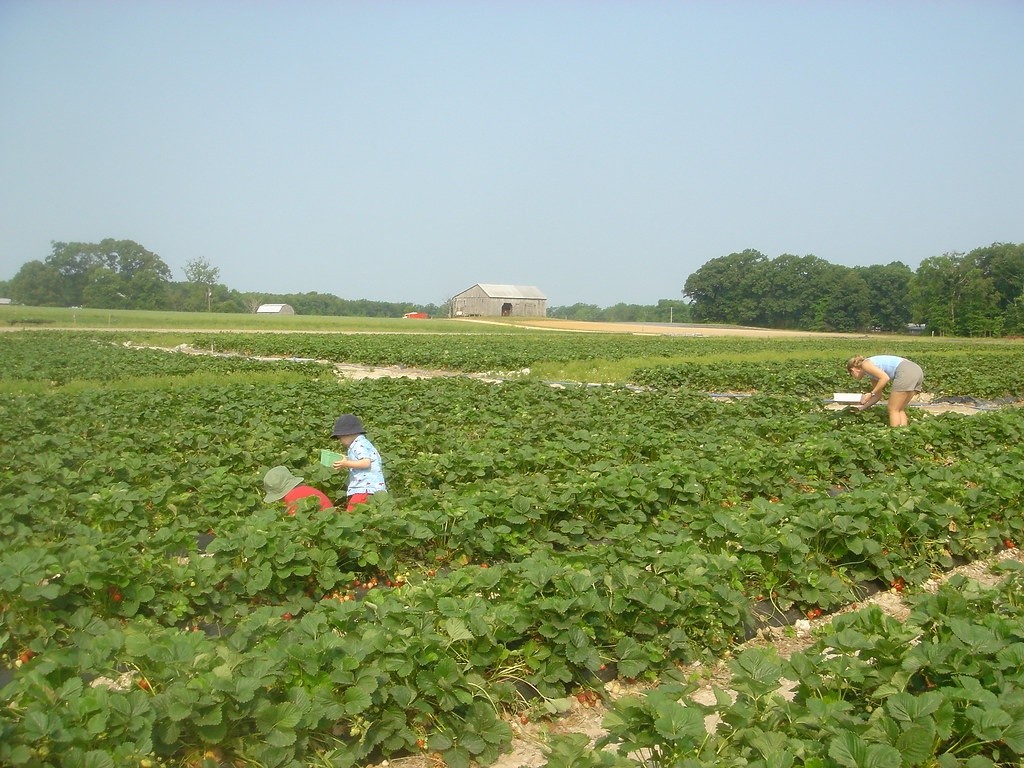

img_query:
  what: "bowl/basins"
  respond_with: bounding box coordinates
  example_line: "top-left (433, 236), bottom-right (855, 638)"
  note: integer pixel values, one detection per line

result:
top-left (321, 449), bottom-right (343, 468)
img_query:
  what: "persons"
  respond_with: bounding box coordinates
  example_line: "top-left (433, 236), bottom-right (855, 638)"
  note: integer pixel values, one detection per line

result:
top-left (265, 465), bottom-right (333, 514)
top-left (331, 414), bottom-right (387, 512)
top-left (847, 354), bottom-right (925, 427)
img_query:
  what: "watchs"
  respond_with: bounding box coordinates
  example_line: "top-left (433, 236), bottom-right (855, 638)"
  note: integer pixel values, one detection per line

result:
top-left (871, 391), bottom-right (875, 397)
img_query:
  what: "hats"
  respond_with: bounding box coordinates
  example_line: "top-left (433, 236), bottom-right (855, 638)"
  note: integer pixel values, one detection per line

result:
top-left (330, 414), bottom-right (367, 438)
top-left (263, 465), bottom-right (304, 504)
